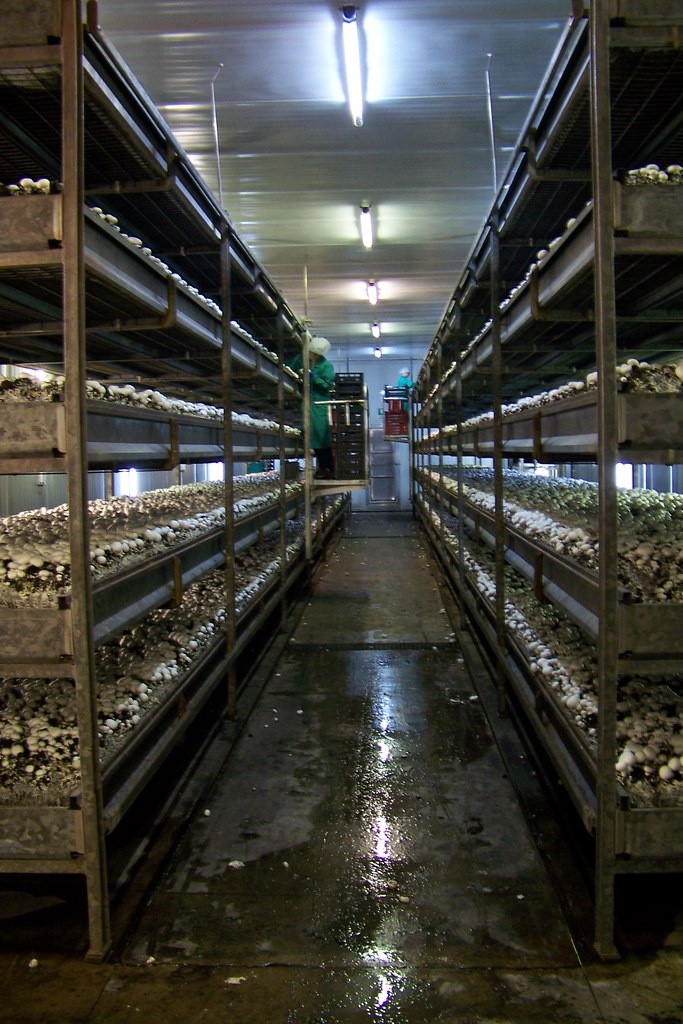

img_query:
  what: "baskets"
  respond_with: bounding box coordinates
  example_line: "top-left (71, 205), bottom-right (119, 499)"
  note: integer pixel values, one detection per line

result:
top-left (384, 384), bottom-right (410, 435)
top-left (333, 372), bottom-right (366, 480)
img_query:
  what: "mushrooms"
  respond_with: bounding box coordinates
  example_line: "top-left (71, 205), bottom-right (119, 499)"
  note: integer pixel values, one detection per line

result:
top-left (416, 162), bottom-right (683, 810)
top-left (0, 178), bottom-right (349, 807)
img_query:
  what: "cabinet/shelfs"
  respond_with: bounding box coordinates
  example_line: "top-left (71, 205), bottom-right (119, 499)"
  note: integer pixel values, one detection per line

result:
top-left (0, 0), bottom-right (351, 960)
top-left (409, 0), bottom-right (682, 963)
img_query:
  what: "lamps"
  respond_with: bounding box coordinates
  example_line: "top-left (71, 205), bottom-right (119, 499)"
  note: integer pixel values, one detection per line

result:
top-left (342, 6), bottom-right (384, 359)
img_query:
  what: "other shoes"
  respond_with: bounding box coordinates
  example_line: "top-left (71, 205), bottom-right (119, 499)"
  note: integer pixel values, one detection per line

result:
top-left (315, 470), bottom-right (328, 478)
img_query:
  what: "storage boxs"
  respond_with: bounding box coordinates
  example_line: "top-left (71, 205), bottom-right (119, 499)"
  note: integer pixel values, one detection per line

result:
top-left (334, 374), bottom-right (365, 480)
top-left (385, 384), bottom-right (407, 435)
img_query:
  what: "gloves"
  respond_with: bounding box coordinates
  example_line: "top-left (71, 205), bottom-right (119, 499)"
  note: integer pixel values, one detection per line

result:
top-left (300, 368), bottom-right (315, 378)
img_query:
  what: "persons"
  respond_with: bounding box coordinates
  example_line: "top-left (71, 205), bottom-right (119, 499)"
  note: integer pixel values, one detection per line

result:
top-left (287, 338), bottom-right (337, 480)
top-left (394, 366), bottom-right (416, 410)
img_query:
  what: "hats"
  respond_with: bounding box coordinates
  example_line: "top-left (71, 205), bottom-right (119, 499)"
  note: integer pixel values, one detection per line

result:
top-left (307, 337), bottom-right (331, 357)
